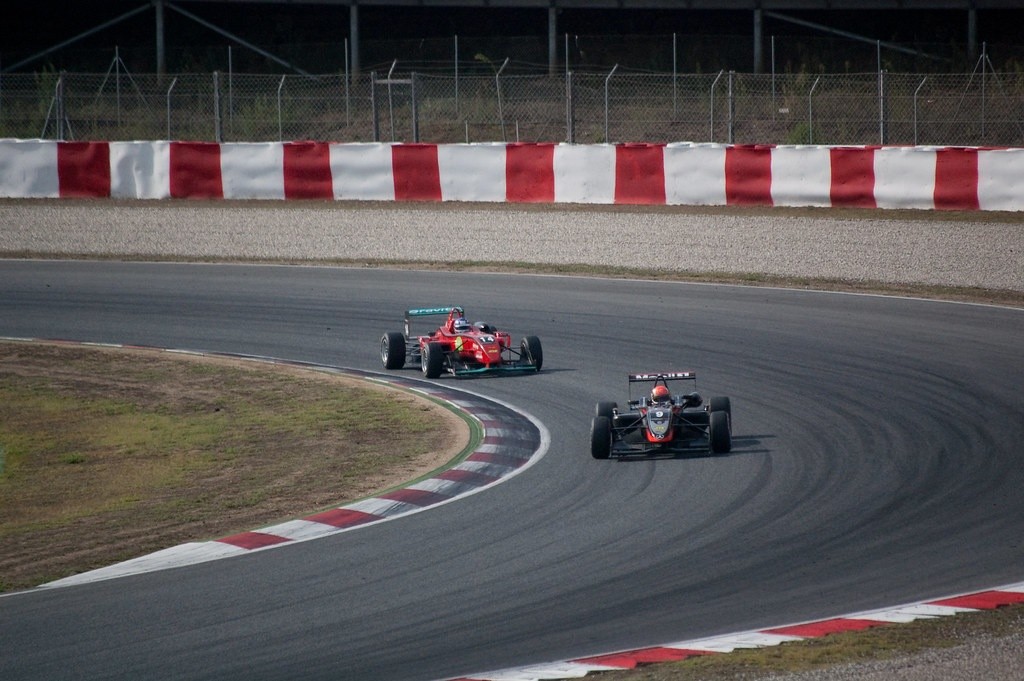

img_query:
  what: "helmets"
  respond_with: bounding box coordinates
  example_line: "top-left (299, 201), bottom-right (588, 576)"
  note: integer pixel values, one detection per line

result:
top-left (454, 317), bottom-right (472, 334)
top-left (651, 386), bottom-right (670, 405)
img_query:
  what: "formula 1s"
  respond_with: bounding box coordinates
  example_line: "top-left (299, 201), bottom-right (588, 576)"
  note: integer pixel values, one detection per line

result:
top-left (589, 372), bottom-right (732, 460)
top-left (378, 306), bottom-right (543, 379)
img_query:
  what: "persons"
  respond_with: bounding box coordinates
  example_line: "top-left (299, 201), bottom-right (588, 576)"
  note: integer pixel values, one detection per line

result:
top-left (651, 386), bottom-right (671, 405)
top-left (453, 318), bottom-right (490, 334)
